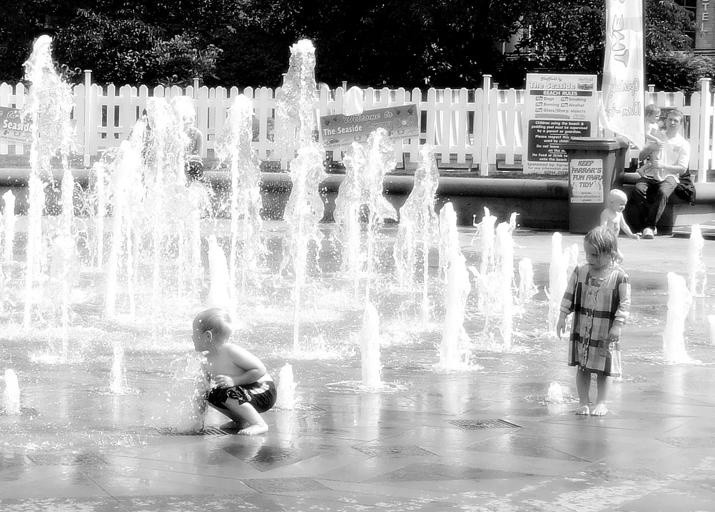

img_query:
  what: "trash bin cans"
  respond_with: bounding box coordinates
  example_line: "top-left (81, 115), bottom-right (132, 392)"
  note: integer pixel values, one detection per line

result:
top-left (559, 136), bottom-right (629, 235)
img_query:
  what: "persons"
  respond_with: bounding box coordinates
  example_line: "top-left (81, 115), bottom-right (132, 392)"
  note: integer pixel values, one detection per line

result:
top-left (599, 187), bottom-right (641, 251)
top-left (186, 126), bottom-right (205, 179)
top-left (556, 225), bottom-right (633, 417)
top-left (644, 102), bottom-right (664, 150)
top-left (191, 306), bottom-right (278, 435)
top-left (631, 107), bottom-right (693, 240)
